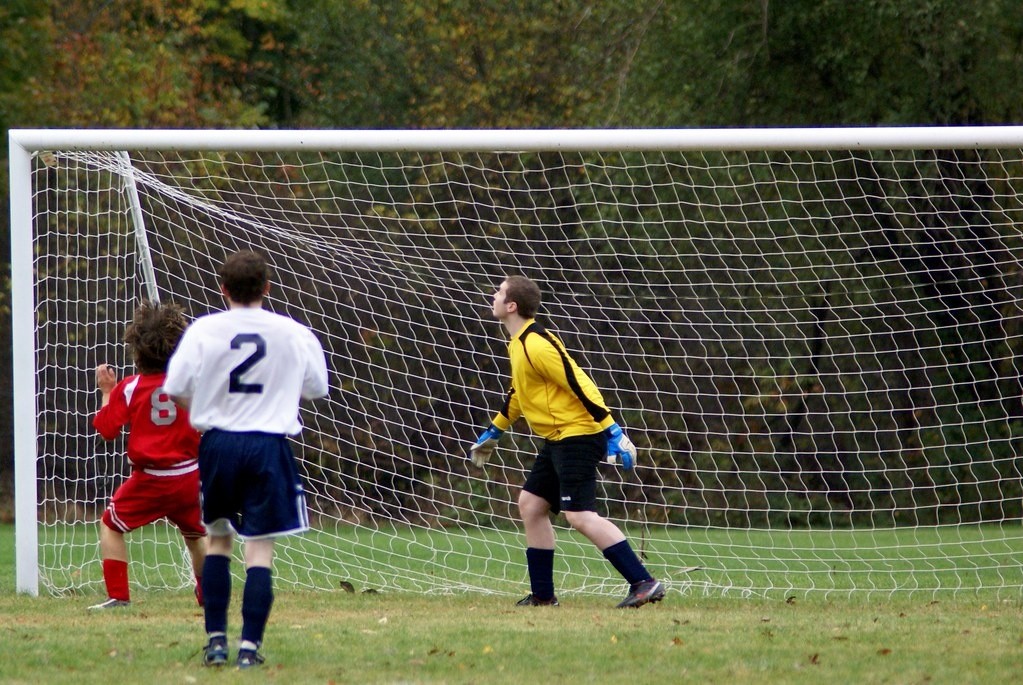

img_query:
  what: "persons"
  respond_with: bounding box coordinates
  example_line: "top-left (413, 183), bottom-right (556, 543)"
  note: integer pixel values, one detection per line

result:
top-left (470, 275), bottom-right (665, 608)
top-left (163, 250), bottom-right (329, 667)
top-left (87, 299), bottom-right (209, 610)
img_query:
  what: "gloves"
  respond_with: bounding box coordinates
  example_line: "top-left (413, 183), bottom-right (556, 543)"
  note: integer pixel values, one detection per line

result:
top-left (605, 422), bottom-right (637, 470)
top-left (470, 424), bottom-right (504, 469)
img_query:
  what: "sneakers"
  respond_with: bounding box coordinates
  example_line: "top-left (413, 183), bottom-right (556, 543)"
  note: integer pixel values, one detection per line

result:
top-left (616, 577), bottom-right (666, 608)
top-left (238, 647), bottom-right (264, 668)
top-left (516, 594), bottom-right (558, 606)
top-left (203, 645), bottom-right (229, 667)
top-left (194, 586), bottom-right (198, 596)
top-left (88, 599), bottom-right (132, 610)
